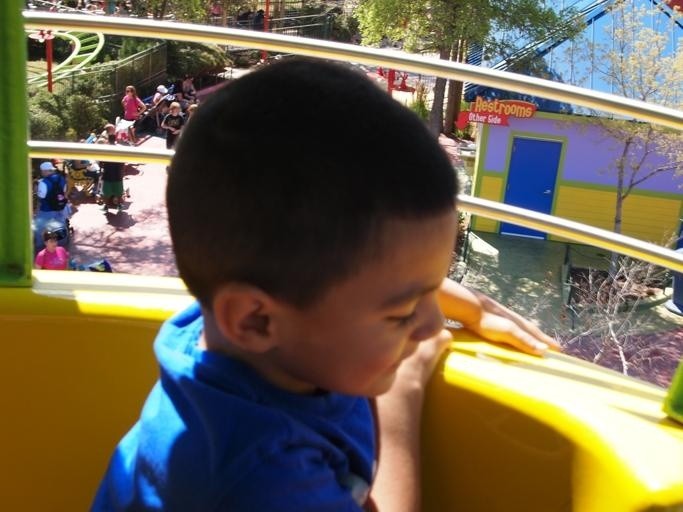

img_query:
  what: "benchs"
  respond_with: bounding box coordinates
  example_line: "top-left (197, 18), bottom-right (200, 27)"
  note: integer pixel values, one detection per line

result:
top-left (139, 84), bottom-right (186, 131)
top-left (62, 133), bottom-right (102, 197)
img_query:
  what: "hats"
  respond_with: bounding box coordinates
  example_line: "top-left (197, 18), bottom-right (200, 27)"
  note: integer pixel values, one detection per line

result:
top-left (156, 84), bottom-right (169, 94)
top-left (39, 161), bottom-right (57, 171)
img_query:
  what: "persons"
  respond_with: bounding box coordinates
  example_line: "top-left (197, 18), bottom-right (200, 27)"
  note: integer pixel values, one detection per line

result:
top-left (210, 2), bottom-right (223, 26)
top-left (27, 0), bottom-right (141, 16)
top-left (31, 64), bottom-right (202, 273)
top-left (90, 52), bottom-right (562, 512)
top-left (254, 9), bottom-right (274, 31)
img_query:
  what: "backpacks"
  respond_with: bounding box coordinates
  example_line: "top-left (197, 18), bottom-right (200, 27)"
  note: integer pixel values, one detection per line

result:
top-left (43, 174), bottom-right (67, 211)
